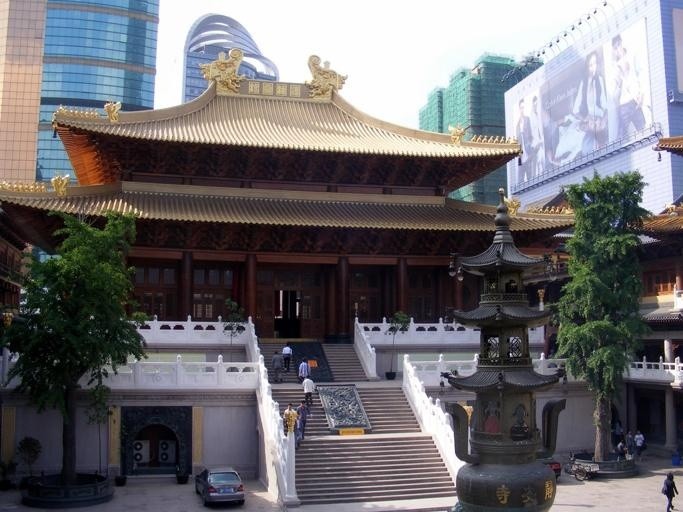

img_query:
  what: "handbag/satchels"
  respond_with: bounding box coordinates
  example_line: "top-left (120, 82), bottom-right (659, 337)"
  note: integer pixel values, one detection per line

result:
top-left (661, 480), bottom-right (668, 495)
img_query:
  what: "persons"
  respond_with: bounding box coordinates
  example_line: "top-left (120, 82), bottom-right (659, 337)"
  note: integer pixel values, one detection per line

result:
top-left (282, 342), bottom-right (293, 372)
top-left (544, 114), bottom-right (607, 169)
top-left (302, 375), bottom-right (314, 410)
top-left (517, 96), bottom-right (543, 185)
top-left (615, 422), bottom-right (645, 460)
top-left (282, 401), bottom-right (312, 449)
top-left (299, 358), bottom-right (310, 380)
top-left (609, 32), bottom-right (648, 141)
top-left (663, 473), bottom-right (678, 512)
top-left (271, 351), bottom-right (283, 383)
top-left (572, 50), bottom-right (607, 160)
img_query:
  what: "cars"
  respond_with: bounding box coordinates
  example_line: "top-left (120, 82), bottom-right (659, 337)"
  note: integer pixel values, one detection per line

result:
top-left (536, 446), bottom-right (561, 476)
top-left (194, 464), bottom-right (245, 506)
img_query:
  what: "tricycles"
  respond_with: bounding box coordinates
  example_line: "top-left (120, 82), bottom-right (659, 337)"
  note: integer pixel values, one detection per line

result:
top-left (565, 448), bottom-right (599, 481)
